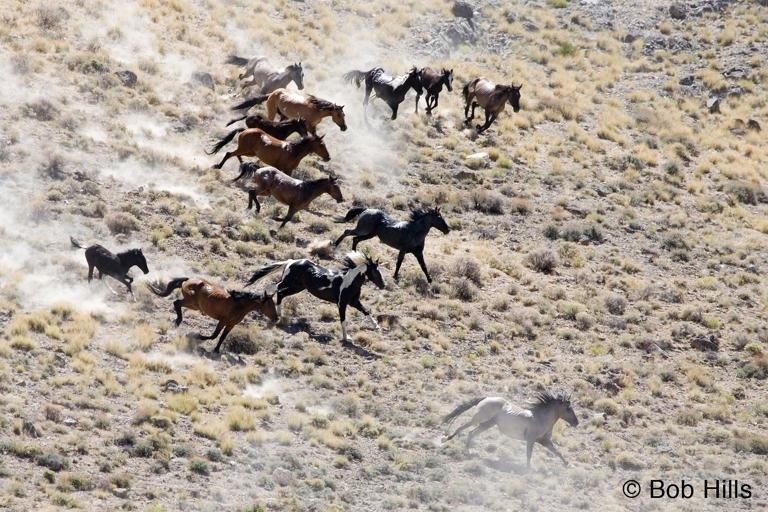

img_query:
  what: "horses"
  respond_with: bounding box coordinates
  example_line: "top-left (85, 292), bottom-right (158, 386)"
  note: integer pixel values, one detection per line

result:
top-left (332, 204), bottom-right (451, 283)
top-left (242, 249), bottom-right (387, 344)
top-left (441, 389), bottom-right (580, 470)
top-left (205, 55), bottom-right (348, 233)
top-left (146, 275), bottom-right (280, 356)
top-left (69, 235), bottom-right (149, 304)
top-left (344, 67), bottom-right (523, 134)
